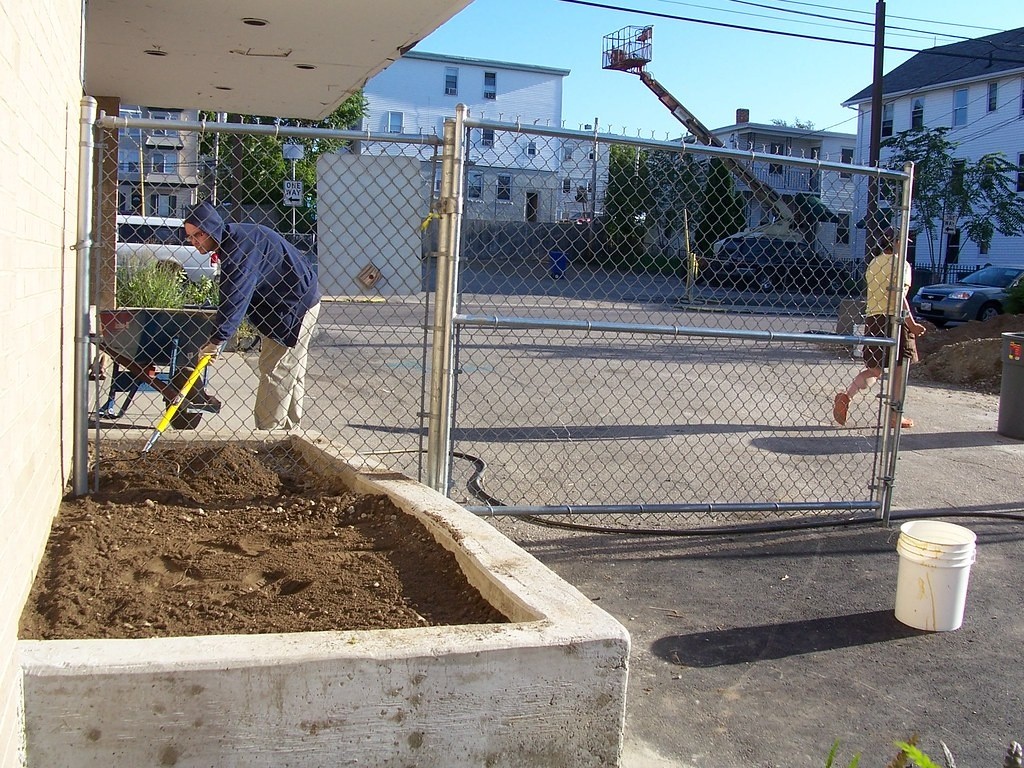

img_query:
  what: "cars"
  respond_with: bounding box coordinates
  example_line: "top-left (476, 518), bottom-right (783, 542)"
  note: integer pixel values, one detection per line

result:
top-left (910, 264), bottom-right (1024, 325)
top-left (710, 238), bottom-right (846, 294)
top-left (594, 212), bottom-right (603, 217)
top-left (553, 210), bottom-right (580, 224)
top-left (577, 212), bottom-right (590, 225)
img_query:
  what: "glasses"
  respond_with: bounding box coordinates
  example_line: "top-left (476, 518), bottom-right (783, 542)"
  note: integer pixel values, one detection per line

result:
top-left (185, 232), bottom-right (205, 242)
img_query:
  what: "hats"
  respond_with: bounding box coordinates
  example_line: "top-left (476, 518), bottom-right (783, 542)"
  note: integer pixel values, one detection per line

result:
top-left (884, 227), bottom-right (913, 243)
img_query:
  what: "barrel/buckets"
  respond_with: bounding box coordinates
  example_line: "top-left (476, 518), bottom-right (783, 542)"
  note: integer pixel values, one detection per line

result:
top-left (894, 520), bottom-right (978, 633)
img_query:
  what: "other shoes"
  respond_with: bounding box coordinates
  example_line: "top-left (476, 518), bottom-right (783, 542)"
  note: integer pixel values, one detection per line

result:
top-left (891, 413), bottom-right (914, 428)
top-left (833, 393), bottom-right (851, 426)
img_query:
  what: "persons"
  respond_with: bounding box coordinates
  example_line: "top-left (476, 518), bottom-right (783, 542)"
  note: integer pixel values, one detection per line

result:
top-left (832, 223), bottom-right (926, 429)
top-left (183, 198), bottom-right (322, 430)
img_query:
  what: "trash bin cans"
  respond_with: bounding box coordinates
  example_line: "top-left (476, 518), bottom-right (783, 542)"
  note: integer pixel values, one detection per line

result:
top-left (549, 251), bottom-right (569, 280)
top-left (997, 332), bottom-right (1024, 441)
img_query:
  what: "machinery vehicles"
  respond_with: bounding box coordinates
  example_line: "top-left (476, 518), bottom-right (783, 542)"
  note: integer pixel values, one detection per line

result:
top-left (602, 23), bottom-right (805, 282)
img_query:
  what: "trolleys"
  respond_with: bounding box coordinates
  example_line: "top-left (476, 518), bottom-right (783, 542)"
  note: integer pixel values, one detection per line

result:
top-left (85, 308), bottom-right (223, 430)
